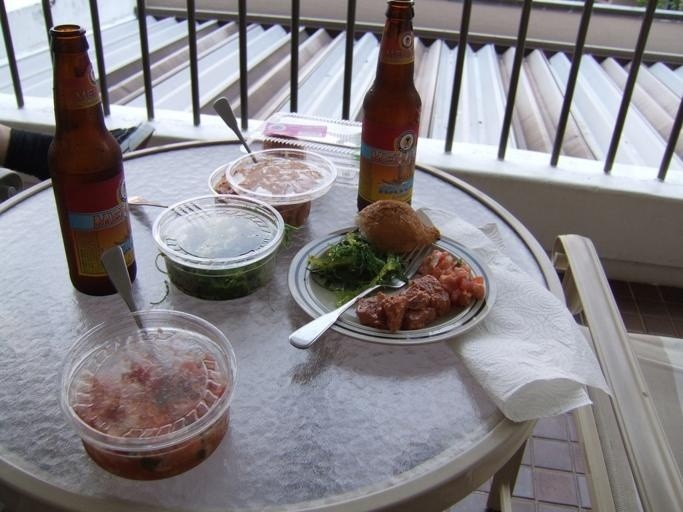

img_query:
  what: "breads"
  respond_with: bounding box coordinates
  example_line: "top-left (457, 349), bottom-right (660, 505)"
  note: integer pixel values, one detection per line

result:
top-left (357, 199), bottom-right (441, 252)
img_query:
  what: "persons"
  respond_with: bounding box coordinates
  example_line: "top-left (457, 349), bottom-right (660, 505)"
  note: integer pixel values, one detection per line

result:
top-left (0, 121), bottom-right (155, 182)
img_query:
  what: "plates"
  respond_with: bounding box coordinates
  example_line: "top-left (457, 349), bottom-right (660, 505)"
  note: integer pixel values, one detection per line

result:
top-left (287, 227), bottom-right (498, 349)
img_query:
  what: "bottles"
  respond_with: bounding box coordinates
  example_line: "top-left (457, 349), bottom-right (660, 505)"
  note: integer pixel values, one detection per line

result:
top-left (358, 1), bottom-right (421, 214)
top-left (47, 23), bottom-right (136, 295)
